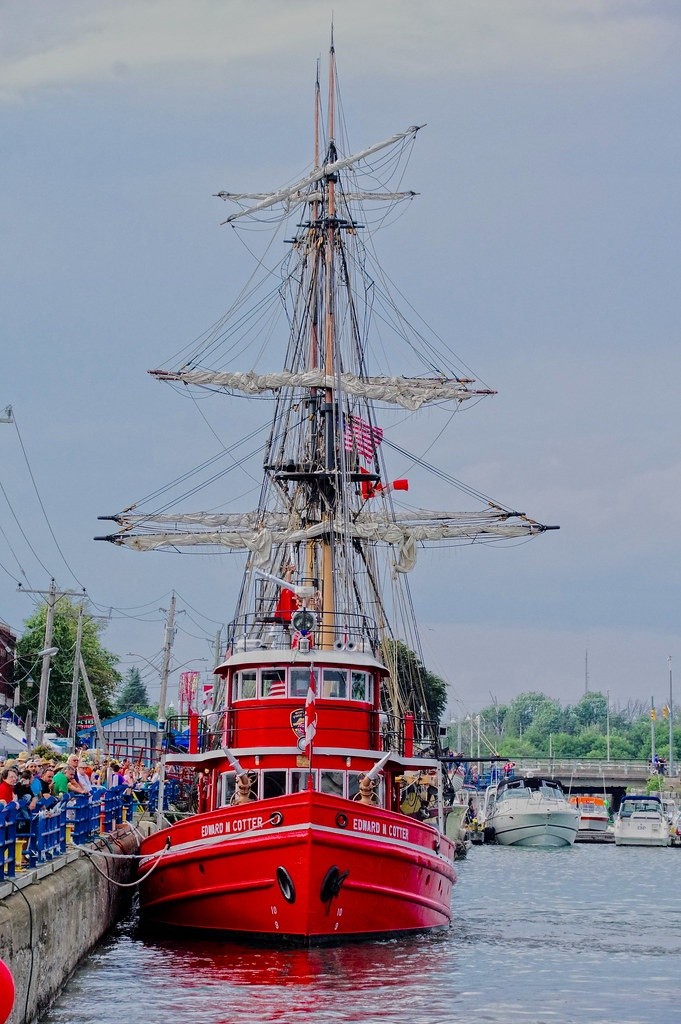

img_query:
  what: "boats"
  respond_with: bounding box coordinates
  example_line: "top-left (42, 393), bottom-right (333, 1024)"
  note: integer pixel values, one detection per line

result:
top-left (421, 753), bottom-right (681, 857)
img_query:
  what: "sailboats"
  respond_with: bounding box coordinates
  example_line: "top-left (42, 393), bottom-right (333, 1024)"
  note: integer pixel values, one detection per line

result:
top-left (91, 23), bottom-right (573, 955)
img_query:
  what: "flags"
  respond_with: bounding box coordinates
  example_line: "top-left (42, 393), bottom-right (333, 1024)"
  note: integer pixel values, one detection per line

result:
top-left (302, 662), bottom-right (319, 759)
top-left (271, 582), bottom-right (303, 624)
top-left (342, 409), bottom-right (410, 499)
top-left (269, 680), bottom-right (285, 697)
top-left (180, 672), bottom-right (213, 708)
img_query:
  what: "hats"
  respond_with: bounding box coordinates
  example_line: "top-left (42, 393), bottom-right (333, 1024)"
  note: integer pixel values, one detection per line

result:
top-left (0, 751), bottom-right (158, 788)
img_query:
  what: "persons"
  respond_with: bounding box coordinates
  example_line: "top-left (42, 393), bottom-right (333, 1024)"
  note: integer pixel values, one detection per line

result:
top-left (648, 754), bottom-right (671, 776)
top-left (0, 747), bottom-right (171, 865)
top-left (447, 747), bottom-right (516, 824)
